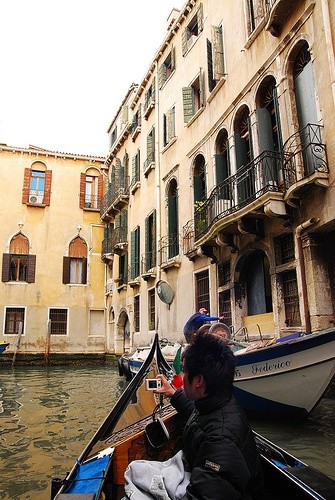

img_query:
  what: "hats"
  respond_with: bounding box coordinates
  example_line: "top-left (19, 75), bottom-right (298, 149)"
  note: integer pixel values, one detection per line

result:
top-left (200, 308), bottom-right (207, 311)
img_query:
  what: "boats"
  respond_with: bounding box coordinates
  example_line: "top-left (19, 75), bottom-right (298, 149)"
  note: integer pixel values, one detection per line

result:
top-left (116, 322), bottom-right (335, 415)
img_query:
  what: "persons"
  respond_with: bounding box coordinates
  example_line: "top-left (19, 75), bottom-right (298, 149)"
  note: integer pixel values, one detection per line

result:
top-left (125, 334), bottom-right (267, 500)
top-left (183, 308), bottom-right (225, 343)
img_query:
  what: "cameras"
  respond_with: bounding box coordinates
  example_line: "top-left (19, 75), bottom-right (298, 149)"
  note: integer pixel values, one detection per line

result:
top-left (145, 378), bottom-right (162, 391)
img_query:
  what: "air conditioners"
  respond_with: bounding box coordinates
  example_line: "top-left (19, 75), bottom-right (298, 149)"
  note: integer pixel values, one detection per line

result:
top-left (208, 199), bottom-right (234, 226)
top-left (29, 195), bottom-right (43, 204)
